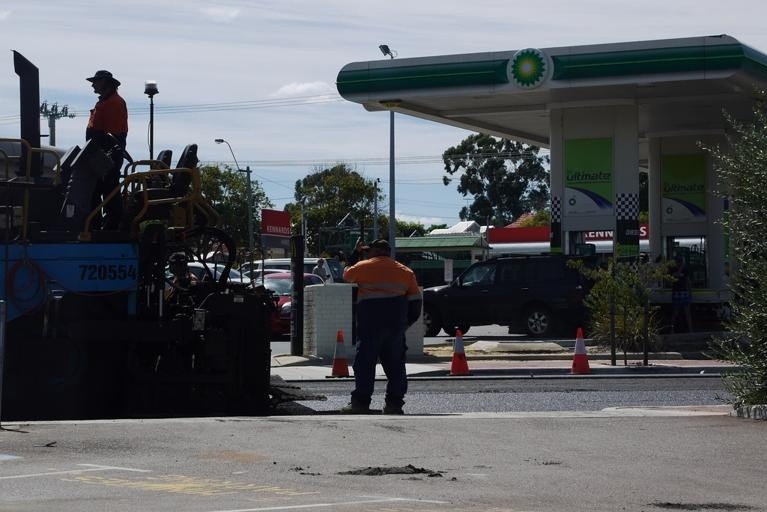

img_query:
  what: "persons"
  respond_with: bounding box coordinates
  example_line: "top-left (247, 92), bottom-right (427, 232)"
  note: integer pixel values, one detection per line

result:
top-left (163, 252), bottom-right (198, 305)
top-left (311, 258), bottom-right (330, 284)
top-left (86, 69), bottom-right (128, 231)
top-left (341, 237), bottom-right (422, 415)
top-left (664, 255), bottom-right (693, 334)
top-left (639, 252), bottom-right (658, 325)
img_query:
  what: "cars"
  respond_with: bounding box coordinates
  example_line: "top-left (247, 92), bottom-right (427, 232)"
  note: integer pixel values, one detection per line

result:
top-left (156, 257), bottom-right (347, 339)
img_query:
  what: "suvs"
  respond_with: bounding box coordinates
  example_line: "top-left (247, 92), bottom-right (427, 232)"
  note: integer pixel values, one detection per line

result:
top-left (421, 251), bottom-right (593, 339)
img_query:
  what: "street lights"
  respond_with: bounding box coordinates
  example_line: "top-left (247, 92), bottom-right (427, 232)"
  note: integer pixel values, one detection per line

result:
top-left (143, 84), bottom-right (160, 161)
top-left (378, 44), bottom-right (397, 262)
top-left (214, 139), bottom-right (256, 257)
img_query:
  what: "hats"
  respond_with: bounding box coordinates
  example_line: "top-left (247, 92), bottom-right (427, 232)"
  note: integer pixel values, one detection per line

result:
top-left (87, 71), bottom-right (121, 85)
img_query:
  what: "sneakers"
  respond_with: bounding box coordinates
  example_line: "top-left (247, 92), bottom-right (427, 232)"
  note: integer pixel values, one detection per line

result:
top-left (342, 404), bottom-right (404, 414)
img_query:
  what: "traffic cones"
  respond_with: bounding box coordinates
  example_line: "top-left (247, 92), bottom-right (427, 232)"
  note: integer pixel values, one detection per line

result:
top-left (326, 329), bottom-right (357, 378)
top-left (569, 327), bottom-right (595, 375)
top-left (446, 329), bottom-right (476, 376)
